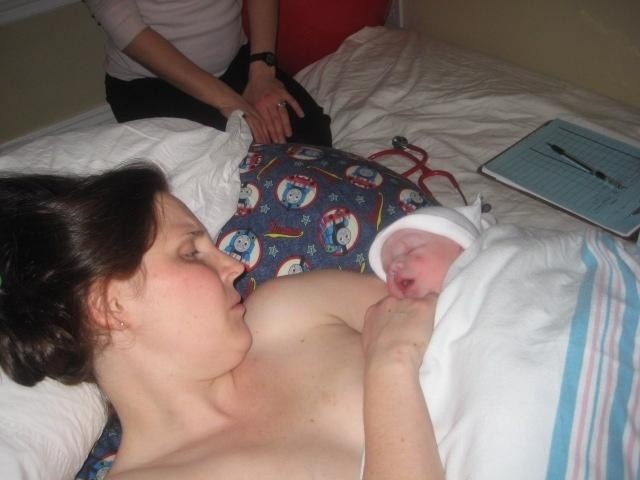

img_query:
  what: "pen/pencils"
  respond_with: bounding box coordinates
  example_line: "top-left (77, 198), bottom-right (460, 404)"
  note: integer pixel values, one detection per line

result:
top-left (545, 141), bottom-right (622, 190)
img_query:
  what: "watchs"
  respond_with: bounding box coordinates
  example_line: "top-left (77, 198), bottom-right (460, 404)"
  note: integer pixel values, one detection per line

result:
top-left (246, 51), bottom-right (278, 66)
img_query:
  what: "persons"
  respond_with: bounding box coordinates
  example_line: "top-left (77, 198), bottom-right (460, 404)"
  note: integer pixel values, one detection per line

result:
top-left (366, 191), bottom-right (480, 301)
top-left (0, 162), bottom-right (447, 480)
top-left (86, 0), bottom-right (333, 147)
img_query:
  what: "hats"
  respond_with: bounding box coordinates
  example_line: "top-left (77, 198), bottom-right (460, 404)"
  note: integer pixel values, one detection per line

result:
top-left (369, 194), bottom-right (497, 283)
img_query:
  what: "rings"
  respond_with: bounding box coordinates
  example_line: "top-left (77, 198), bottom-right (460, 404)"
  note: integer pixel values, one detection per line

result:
top-left (276, 100), bottom-right (287, 109)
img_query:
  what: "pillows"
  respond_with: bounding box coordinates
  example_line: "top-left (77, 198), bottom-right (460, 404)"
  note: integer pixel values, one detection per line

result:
top-left (79, 138), bottom-right (443, 478)
top-left (0, 110), bottom-right (252, 478)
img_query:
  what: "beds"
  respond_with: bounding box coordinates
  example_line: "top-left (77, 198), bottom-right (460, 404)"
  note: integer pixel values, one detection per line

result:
top-left (0, 25), bottom-right (639, 479)
